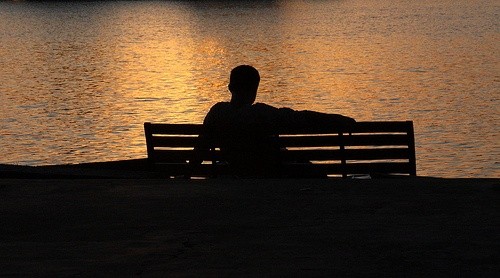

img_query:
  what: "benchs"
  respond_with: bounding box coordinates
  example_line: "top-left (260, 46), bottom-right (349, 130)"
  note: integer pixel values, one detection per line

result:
top-left (143, 121), bottom-right (416, 178)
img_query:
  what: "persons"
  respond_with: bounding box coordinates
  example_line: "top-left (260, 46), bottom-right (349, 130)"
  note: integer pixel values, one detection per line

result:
top-left (182, 65), bottom-right (357, 178)
